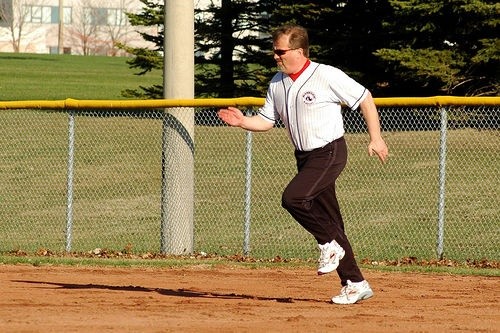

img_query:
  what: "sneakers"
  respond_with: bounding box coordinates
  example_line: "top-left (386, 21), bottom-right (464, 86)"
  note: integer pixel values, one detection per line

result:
top-left (316, 240), bottom-right (345, 275)
top-left (331, 279), bottom-right (374, 305)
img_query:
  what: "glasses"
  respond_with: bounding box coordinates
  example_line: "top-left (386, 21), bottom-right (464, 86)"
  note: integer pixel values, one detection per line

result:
top-left (272, 47), bottom-right (301, 55)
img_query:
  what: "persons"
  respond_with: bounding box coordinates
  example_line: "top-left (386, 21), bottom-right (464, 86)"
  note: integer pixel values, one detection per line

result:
top-left (217, 26), bottom-right (390, 306)
